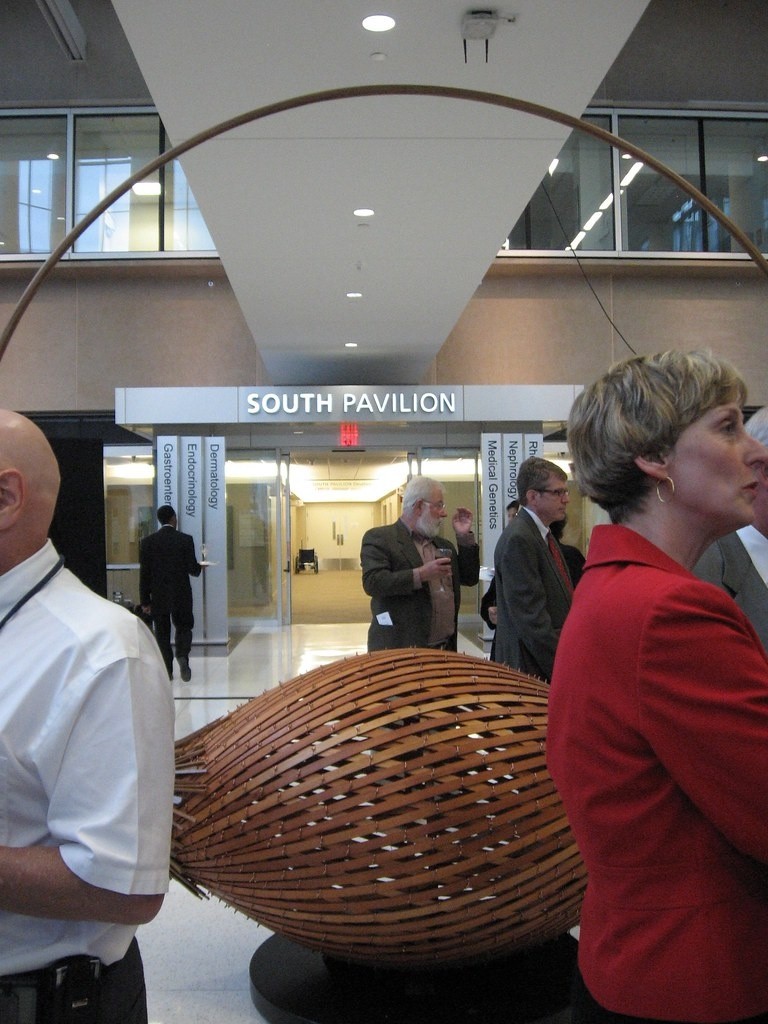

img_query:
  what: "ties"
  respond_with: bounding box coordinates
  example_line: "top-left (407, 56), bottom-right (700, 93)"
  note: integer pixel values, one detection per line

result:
top-left (547, 529), bottom-right (573, 595)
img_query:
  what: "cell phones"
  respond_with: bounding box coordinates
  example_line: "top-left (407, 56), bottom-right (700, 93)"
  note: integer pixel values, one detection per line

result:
top-left (435, 548), bottom-right (453, 560)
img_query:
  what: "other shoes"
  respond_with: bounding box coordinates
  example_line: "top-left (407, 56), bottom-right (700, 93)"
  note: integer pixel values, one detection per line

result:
top-left (178, 656), bottom-right (191, 681)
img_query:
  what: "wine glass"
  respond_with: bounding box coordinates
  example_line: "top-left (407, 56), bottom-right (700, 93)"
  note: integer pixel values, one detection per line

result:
top-left (200, 544), bottom-right (209, 562)
top-left (433, 549), bottom-right (452, 593)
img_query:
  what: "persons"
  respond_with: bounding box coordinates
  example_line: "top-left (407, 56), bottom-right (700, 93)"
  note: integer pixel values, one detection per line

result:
top-left (0, 409), bottom-right (175, 1024)
top-left (691, 407), bottom-right (768, 656)
top-left (481, 456), bottom-right (587, 684)
top-left (360, 475), bottom-right (480, 653)
top-left (545, 350), bottom-right (768, 1024)
top-left (139, 505), bottom-right (208, 682)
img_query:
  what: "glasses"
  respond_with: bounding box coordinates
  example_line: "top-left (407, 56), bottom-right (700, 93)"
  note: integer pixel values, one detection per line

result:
top-left (412, 500), bottom-right (445, 510)
top-left (534, 488), bottom-right (570, 498)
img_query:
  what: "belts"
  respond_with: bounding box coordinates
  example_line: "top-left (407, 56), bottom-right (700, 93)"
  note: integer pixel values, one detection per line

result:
top-left (433, 642), bottom-right (453, 650)
